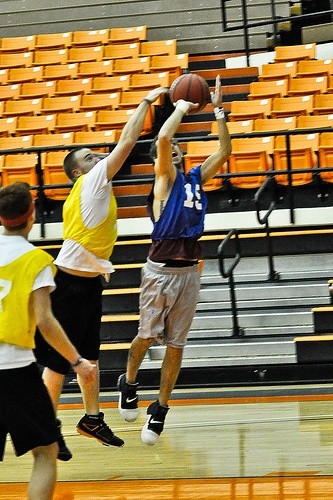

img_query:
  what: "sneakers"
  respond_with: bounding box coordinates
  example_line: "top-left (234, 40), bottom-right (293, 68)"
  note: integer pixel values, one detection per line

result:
top-left (141, 402), bottom-right (169, 445)
top-left (77, 409), bottom-right (124, 447)
top-left (54, 419), bottom-right (71, 461)
top-left (116, 374), bottom-right (140, 422)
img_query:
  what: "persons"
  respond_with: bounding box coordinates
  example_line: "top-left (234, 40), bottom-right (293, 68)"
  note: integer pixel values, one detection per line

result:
top-left (0, 184), bottom-right (96, 500)
top-left (117, 74), bottom-right (232, 446)
top-left (35, 87), bottom-right (167, 461)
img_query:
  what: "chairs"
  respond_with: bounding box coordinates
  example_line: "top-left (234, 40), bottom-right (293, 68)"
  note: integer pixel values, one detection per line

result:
top-left (0, 25), bottom-right (333, 200)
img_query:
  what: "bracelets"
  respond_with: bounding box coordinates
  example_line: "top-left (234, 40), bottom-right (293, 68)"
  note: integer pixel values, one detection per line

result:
top-left (214, 107), bottom-right (224, 119)
top-left (74, 357), bottom-right (82, 366)
top-left (142, 98), bottom-right (151, 104)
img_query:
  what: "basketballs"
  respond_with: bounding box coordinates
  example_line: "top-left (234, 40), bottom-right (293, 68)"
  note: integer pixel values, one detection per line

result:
top-left (170, 74), bottom-right (209, 114)
